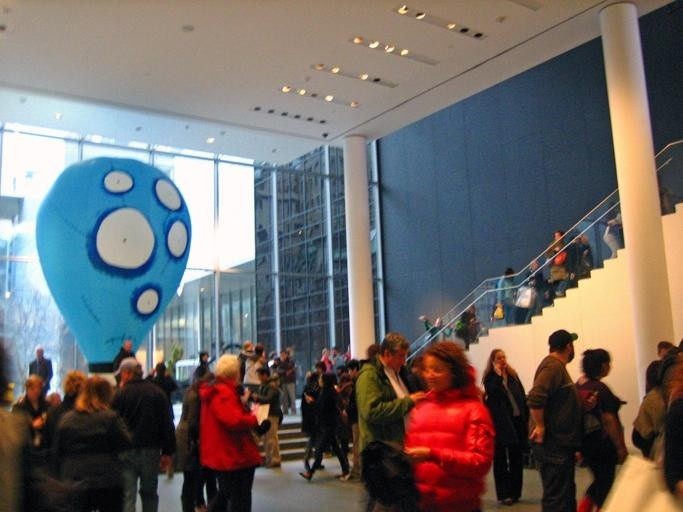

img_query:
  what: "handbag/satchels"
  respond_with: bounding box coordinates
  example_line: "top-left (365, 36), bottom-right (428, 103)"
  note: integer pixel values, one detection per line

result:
top-left (514, 281), bottom-right (538, 310)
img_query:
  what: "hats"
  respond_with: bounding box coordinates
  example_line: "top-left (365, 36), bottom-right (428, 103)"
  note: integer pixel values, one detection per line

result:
top-left (112, 357), bottom-right (142, 377)
top-left (547, 329), bottom-right (578, 354)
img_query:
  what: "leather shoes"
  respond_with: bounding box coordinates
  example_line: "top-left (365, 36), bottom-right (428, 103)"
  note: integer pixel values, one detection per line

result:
top-left (502, 498), bottom-right (519, 506)
top-left (259, 461), bottom-right (350, 482)
